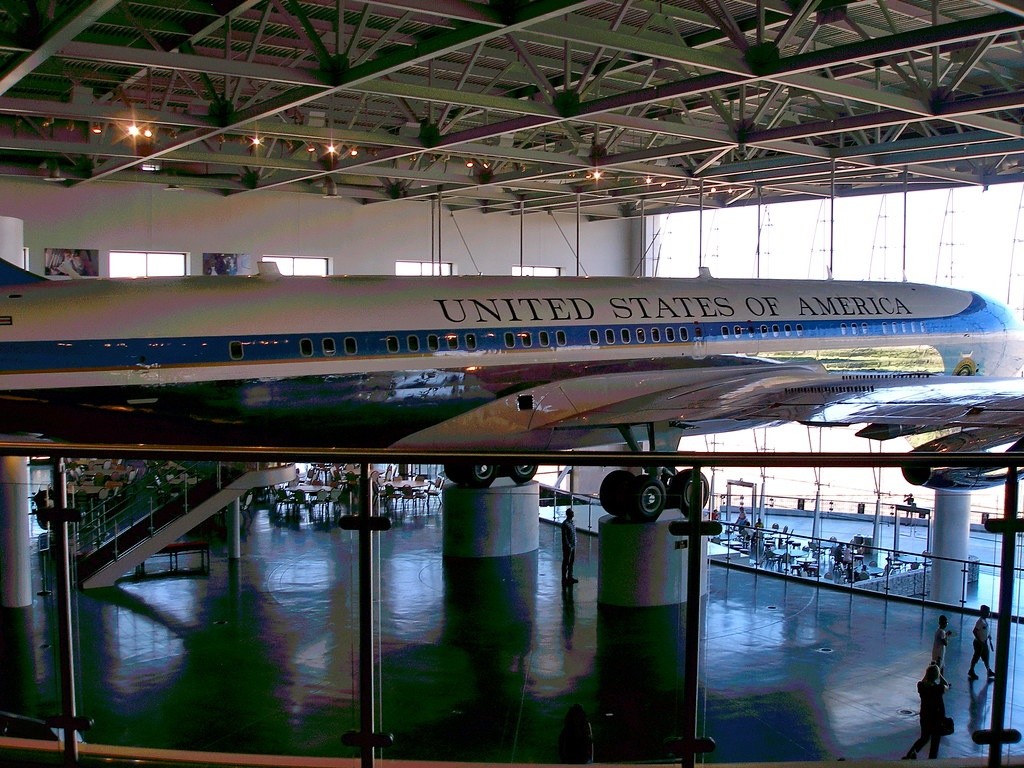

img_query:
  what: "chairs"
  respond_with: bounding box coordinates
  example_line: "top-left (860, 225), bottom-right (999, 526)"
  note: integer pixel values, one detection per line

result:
top-left (34, 458), bottom-right (447, 526)
top-left (711, 515), bottom-right (933, 585)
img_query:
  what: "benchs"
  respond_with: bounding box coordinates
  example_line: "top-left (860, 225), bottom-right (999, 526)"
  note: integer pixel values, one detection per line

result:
top-left (131, 540), bottom-right (210, 577)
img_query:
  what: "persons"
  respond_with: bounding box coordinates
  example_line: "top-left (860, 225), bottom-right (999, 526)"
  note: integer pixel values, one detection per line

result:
top-left (904, 493), bottom-right (914, 517)
top-left (968, 605), bottom-right (996, 680)
top-left (835, 542), bottom-right (854, 575)
top-left (711, 507), bottom-right (765, 539)
top-left (560, 507), bottom-right (579, 589)
top-left (901, 665), bottom-right (955, 759)
top-left (63, 458), bottom-right (126, 488)
top-left (933, 615), bottom-right (952, 690)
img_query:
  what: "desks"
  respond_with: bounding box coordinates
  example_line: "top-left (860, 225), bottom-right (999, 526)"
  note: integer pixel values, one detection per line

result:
top-left (772, 548), bottom-right (809, 571)
top-left (342, 468), bottom-right (385, 482)
top-left (721, 540), bottom-right (744, 550)
top-left (815, 540), bottom-right (836, 556)
top-left (382, 480), bottom-right (428, 508)
top-left (86, 469), bottom-right (128, 483)
top-left (67, 484), bottom-right (110, 522)
top-left (74, 459), bottom-right (114, 469)
top-left (854, 564), bottom-right (883, 578)
top-left (283, 485), bottom-right (334, 514)
top-left (796, 557), bottom-right (816, 571)
top-left (898, 554), bottom-right (931, 570)
top-left (770, 529), bottom-right (792, 549)
top-left (712, 532), bottom-right (736, 541)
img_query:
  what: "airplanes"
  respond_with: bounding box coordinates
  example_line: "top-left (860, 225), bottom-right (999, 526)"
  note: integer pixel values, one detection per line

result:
top-left (0, 256), bottom-right (1024, 524)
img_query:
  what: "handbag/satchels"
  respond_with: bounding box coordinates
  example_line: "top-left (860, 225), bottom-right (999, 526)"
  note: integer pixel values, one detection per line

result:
top-left (935, 717), bottom-right (954, 736)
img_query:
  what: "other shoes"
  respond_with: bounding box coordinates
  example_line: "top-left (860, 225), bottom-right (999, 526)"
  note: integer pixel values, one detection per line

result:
top-left (906, 747), bottom-right (917, 759)
top-left (563, 576), bottom-right (579, 584)
top-left (987, 669), bottom-right (995, 676)
top-left (968, 669), bottom-right (978, 680)
